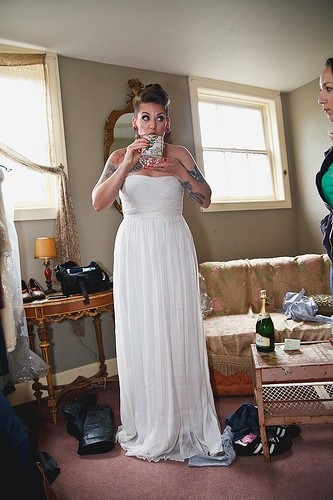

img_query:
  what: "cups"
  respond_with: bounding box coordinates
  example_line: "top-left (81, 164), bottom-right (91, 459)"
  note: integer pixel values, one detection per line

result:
top-left (140, 134), bottom-right (164, 164)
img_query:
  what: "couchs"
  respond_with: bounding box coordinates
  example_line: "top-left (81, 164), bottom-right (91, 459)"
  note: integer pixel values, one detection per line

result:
top-left (197, 254), bottom-right (333, 395)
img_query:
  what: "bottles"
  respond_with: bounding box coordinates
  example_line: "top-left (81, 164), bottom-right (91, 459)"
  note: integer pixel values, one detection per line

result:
top-left (256, 289), bottom-right (275, 353)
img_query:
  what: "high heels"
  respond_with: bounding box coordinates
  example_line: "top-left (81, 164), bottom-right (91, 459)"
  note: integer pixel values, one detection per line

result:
top-left (21, 278), bottom-right (46, 303)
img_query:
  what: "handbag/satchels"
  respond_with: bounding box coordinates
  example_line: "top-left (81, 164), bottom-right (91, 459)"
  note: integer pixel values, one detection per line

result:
top-left (59, 261), bottom-right (110, 305)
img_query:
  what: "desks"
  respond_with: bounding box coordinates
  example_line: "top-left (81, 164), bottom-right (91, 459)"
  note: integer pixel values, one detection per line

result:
top-left (250, 341), bottom-right (333, 462)
top-left (16, 290), bottom-right (125, 428)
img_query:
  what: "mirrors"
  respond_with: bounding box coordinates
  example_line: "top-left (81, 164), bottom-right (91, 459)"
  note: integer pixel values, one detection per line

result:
top-left (101, 78), bottom-right (171, 217)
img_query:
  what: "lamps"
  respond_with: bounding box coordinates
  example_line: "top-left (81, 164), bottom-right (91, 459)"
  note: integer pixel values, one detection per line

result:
top-left (34, 238), bottom-right (59, 294)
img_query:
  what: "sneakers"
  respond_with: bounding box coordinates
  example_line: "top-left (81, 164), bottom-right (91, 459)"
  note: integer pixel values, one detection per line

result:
top-left (232, 424), bottom-right (300, 456)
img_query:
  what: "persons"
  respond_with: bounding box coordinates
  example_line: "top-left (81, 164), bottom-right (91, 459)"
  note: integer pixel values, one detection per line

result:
top-left (315, 58), bottom-right (333, 293)
top-left (92, 84), bottom-right (224, 462)
